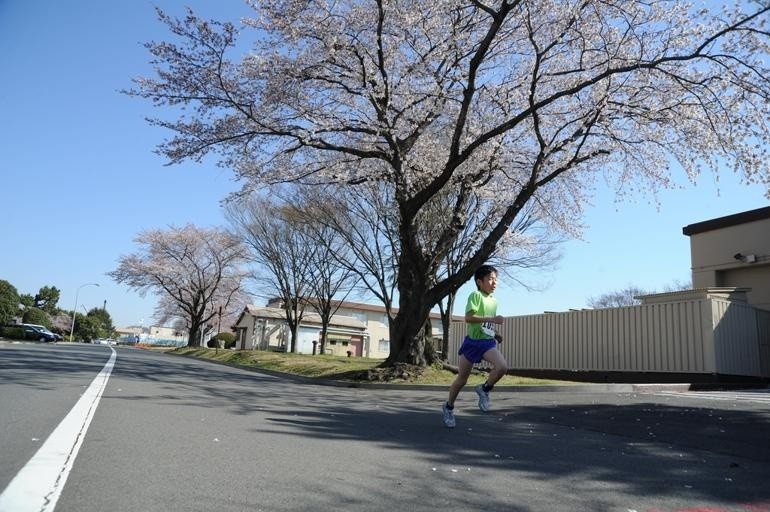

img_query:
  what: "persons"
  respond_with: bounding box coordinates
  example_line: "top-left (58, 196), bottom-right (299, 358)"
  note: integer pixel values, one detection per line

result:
top-left (442, 265), bottom-right (507, 428)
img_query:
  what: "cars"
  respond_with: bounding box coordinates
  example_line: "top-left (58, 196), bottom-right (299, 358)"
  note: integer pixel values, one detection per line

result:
top-left (95, 338), bottom-right (118, 346)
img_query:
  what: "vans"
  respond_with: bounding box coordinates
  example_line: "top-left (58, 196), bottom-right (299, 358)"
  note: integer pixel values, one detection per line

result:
top-left (0, 323), bottom-right (64, 343)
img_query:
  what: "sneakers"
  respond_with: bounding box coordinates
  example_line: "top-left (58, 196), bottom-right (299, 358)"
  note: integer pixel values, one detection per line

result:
top-left (474, 383), bottom-right (492, 412)
top-left (441, 400), bottom-right (456, 428)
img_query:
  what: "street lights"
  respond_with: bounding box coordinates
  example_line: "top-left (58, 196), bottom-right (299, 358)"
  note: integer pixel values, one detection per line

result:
top-left (68, 282), bottom-right (100, 344)
